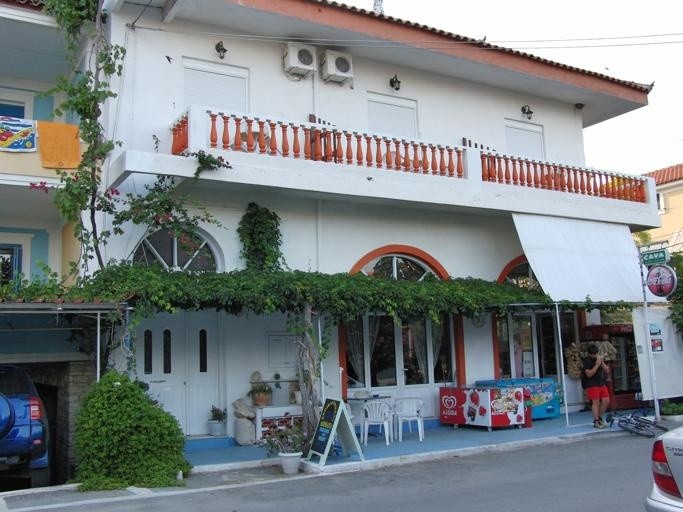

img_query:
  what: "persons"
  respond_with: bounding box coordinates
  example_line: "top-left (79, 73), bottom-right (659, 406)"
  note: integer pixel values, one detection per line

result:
top-left (580, 344), bottom-right (611, 429)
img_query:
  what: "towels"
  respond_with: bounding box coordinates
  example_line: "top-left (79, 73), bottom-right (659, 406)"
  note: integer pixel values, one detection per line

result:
top-left (0, 115), bottom-right (82, 170)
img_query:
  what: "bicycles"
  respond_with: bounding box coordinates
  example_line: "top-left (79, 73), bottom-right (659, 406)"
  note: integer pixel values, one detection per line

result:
top-left (606, 410), bottom-right (668, 437)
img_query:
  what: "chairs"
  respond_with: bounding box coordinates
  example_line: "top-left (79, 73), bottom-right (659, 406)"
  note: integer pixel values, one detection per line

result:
top-left (344, 397), bottom-right (425, 447)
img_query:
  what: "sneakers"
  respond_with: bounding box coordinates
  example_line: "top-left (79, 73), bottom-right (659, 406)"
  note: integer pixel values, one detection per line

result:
top-left (594, 420), bottom-right (608, 428)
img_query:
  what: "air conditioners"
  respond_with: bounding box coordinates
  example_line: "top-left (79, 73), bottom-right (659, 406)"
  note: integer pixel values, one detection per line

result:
top-left (283, 41), bottom-right (354, 84)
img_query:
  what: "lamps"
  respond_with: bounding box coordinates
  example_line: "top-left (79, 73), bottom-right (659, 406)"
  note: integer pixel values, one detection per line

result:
top-left (521, 104), bottom-right (533, 120)
top-left (389, 74), bottom-right (402, 90)
top-left (215, 41), bottom-right (228, 60)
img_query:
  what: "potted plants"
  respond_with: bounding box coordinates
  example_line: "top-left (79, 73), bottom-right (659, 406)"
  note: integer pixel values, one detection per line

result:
top-left (246, 383), bottom-right (274, 406)
top-left (294, 368), bottom-right (320, 405)
top-left (257, 412), bottom-right (310, 474)
top-left (207, 404), bottom-right (227, 436)
top-left (0, 257), bottom-right (118, 303)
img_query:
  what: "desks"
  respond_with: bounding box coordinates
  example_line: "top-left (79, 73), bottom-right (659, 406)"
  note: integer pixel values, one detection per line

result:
top-left (249, 404), bottom-right (323, 445)
top-left (346, 395), bottom-right (391, 438)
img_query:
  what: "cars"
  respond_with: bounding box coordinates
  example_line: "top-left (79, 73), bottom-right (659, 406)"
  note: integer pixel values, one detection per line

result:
top-left (646, 426), bottom-right (683, 512)
top-left (0, 364), bottom-right (52, 488)
